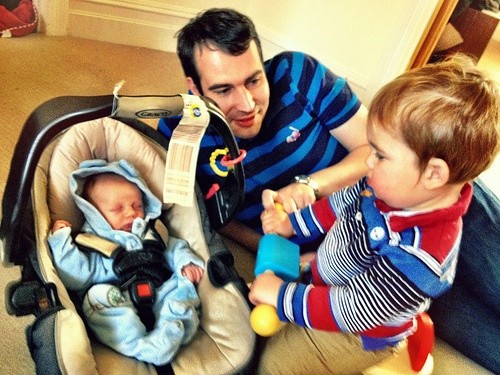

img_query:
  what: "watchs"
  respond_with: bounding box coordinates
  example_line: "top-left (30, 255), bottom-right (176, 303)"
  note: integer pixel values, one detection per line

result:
top-left (290, 174), bottom-right (320, 200)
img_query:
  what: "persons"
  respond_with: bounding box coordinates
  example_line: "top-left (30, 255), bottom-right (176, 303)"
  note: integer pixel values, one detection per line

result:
top-left (246, 51), bottom-right (500, 375)
top-left (45, 159), bottom-right (206, 366)
top-left (154, 7), bottom-right (500, 375)
